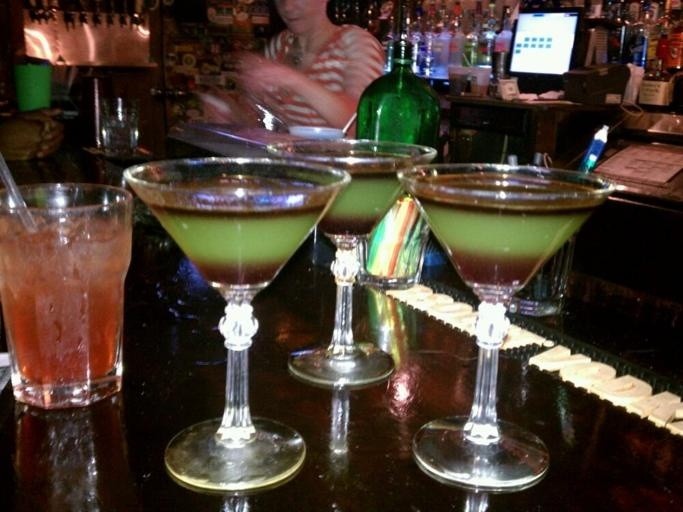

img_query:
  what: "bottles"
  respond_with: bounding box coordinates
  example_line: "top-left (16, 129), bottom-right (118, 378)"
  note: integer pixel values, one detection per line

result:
top-left (354, 40), bottom-right (442, 157)
top-left (329, 3), bottom-right (682, 110)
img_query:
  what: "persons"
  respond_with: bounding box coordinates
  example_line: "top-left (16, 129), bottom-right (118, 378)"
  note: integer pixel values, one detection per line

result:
top-left (67, 66), bottom-right (104, 121)
top-left (195, 0), bottom-right (386, 139)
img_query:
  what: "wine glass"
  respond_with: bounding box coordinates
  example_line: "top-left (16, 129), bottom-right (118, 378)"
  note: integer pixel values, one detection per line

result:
top-left (124, 157), bottom-right (351, 497)
top-left (395, 162), bottom-right (614, 492)
top-left (270, 139), bottom-right (437, 392)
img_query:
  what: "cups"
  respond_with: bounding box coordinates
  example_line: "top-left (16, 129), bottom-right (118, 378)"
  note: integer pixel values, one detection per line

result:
top-left (511, 228), bottom-right (583, 315)
top-left (355, 192), bottom-right (429, 292)
top-left (0, 183), bottom-right (133, 409)
top-left (97, 99), bottom-right (139, 154)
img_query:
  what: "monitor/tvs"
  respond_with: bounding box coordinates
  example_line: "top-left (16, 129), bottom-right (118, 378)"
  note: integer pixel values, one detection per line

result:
top-left (506, 7), bottom-right (591, 76)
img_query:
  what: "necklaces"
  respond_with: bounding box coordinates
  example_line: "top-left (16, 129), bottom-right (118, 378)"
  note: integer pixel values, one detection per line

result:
top-left (293, 36), bottom-right (304, 65)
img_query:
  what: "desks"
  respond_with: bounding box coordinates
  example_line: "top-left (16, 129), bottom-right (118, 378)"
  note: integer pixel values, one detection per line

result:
top-left (444, 97), bottom-right (581, 166)
